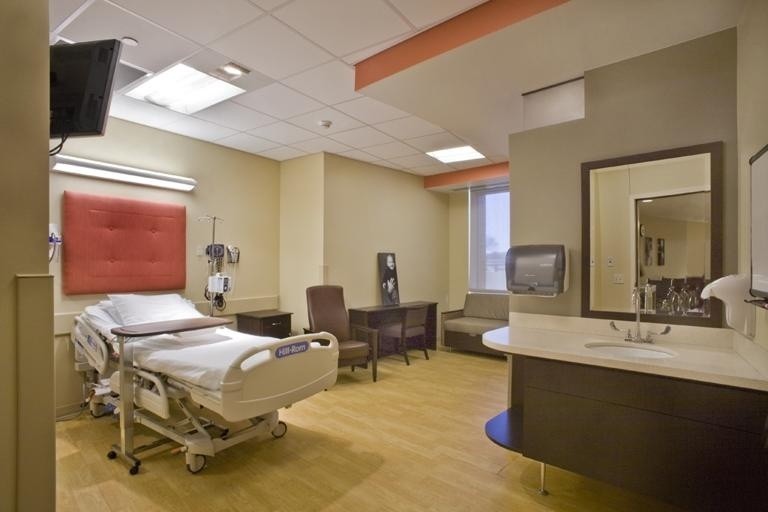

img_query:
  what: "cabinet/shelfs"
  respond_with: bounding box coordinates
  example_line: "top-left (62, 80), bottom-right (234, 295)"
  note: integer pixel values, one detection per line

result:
top-left (235, 309), bottom-right (294, 338)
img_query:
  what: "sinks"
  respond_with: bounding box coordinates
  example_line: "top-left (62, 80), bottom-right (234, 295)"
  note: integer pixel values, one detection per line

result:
top-left (583, 338), bottom-right (679, 362)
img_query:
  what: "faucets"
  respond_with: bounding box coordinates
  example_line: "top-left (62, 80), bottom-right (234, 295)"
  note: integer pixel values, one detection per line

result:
top-left (630, 285), bottom-right (643, 341)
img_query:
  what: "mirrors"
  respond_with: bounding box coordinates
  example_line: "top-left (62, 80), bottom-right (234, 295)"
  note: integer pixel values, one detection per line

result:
top-left (580, 140), bottom-right (723, 328)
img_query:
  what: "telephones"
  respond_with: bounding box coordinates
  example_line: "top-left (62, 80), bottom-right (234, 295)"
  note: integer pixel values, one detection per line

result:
top-left (226, 246), bottom-right (240, 264)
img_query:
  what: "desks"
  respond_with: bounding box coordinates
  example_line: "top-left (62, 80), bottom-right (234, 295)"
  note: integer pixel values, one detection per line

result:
top-left (348, 300), bottom-right (439, 355)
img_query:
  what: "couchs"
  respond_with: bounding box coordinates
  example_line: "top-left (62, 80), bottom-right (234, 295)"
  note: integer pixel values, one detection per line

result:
top-left (441, 291), bottom-right (510, 360)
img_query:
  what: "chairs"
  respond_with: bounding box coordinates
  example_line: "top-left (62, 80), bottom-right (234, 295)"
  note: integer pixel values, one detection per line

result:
top-left (377, 305), bottom-right (430, 366)
top-left (303, 285), bottom-right (379, 391)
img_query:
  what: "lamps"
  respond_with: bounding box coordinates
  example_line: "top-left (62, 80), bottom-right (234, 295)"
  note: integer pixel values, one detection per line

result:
top-left (50, 153), bottom-right (197, 192)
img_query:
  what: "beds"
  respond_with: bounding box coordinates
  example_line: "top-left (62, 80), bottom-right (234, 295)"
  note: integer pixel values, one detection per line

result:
top-left (70, 293), bottom-right (339, 473)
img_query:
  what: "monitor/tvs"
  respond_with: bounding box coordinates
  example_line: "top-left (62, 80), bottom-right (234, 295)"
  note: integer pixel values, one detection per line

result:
top-left (50, 39), bottom-right (124, 139)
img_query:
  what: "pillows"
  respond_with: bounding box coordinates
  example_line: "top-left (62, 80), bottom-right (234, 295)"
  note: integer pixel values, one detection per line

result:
top-left (85, 291), bottom-right (206, 344)
